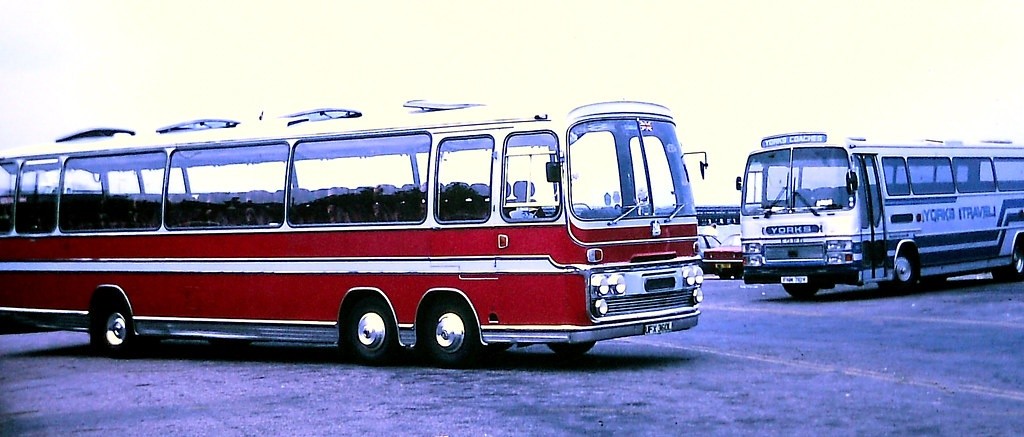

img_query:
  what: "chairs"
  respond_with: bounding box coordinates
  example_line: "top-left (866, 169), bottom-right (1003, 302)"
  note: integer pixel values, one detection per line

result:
top-left (1, 182), bottom-right (534, 238)
top-left (786, 179), bottom-right (1024, 211)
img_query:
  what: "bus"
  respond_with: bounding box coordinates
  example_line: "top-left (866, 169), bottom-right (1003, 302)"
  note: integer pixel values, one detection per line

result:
top-left (1, 93), bottom-right (702, 366)
top-left (692, 204), bottom-right (744, 280)
top-left (736, 133), bottom-right (1024, 295)
